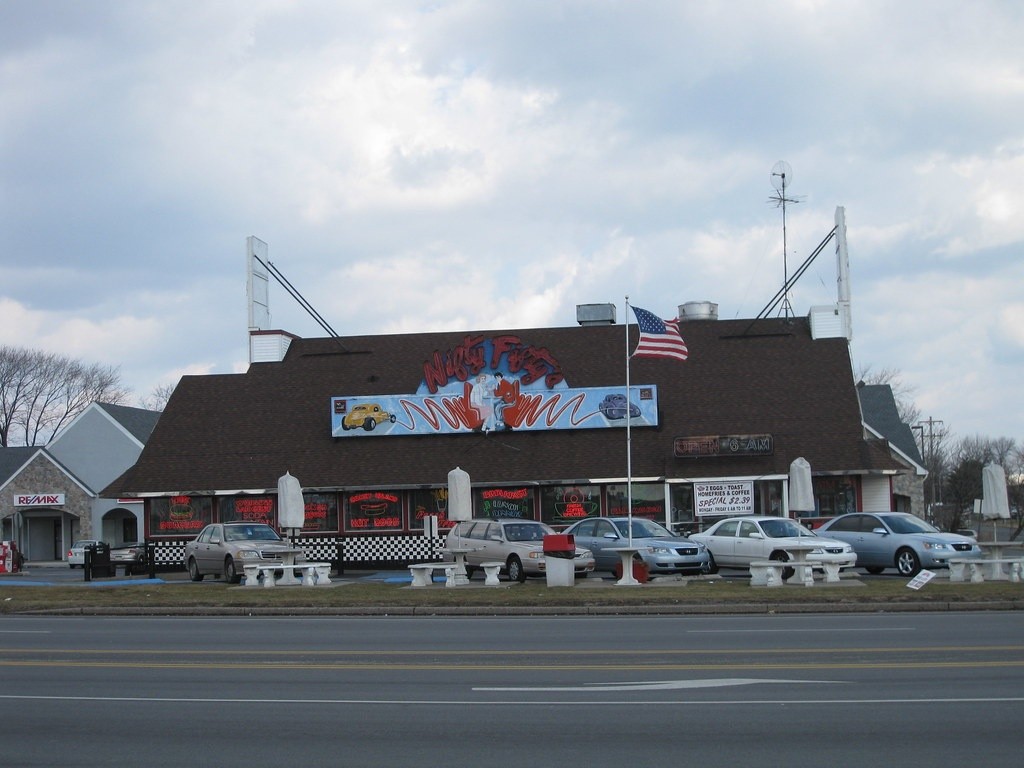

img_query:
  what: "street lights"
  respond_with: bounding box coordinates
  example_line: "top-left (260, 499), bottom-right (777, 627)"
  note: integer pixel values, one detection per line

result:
top-left (911, 426), bottom-right (925, 469)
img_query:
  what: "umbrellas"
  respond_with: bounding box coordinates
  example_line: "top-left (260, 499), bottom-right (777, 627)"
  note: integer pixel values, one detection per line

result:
top-left (277, 468), bottom-right (305, 549)
top-left (788, 456), bottom-right (815, 545)
top-left (981, 459), bottom-right (1011, 543)
top-left (447, 466), bottom-right (472, 550)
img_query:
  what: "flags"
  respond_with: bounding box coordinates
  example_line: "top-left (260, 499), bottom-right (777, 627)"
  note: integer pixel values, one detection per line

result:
top-left (629, 304), bottom-right (689, 363)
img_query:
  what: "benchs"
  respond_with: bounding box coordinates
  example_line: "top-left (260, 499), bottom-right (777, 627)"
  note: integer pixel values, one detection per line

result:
top-left (480, 561), bottom-right (505, 586)
top-left (949, 558), bottom-right (1024, 583)
top-left (243, 562), bottom-right (331, 588)
top-left (749, 558), bottom-right (848, 586)
top-left (408, 561), bottom-right (469, 586)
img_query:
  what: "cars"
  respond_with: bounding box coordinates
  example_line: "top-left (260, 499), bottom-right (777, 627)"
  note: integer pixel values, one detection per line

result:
top-left (560, 515), bottom-right (711, 576)
top-left (927, 502), bottom-right (1023, 527)
top-left (186, 522), bottom-right (307, 582)
top-left (689, 513), bottom-right (859, 578)
top-left (109, 543), bottom-right (150, 565)
top-left (812, 513), bottom-right (985, 574)
top-left (68, 540), bottom-right (111, 568)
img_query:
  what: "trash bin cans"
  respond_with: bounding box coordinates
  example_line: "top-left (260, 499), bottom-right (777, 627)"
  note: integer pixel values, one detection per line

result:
top-left (90, 541), bottom-right (110, 579)
top-left (543, 534), bottom-right (575, 587)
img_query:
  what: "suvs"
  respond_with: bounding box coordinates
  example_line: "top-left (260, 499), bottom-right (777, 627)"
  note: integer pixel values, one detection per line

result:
top-left (444, 517), bottom-right (597, 581)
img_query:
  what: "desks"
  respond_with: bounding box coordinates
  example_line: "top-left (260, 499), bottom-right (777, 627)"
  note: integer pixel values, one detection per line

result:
top-left (774, 545), bottom-right (822, 584)
top-left (435, 548), bottom-right (482, 585)
top-left (601, 547), bottom-right (652, 586)
top-left (263, 548), bottom-right (312, 585)
top-left (969, 541), bottom-right (1023, 580)
